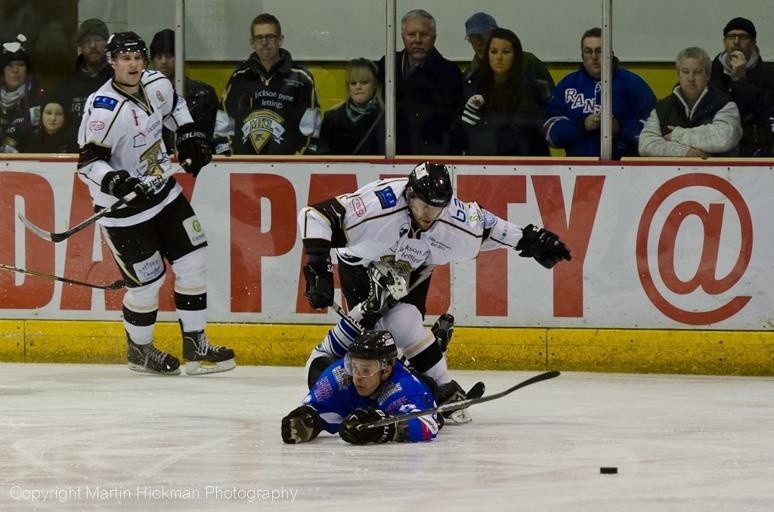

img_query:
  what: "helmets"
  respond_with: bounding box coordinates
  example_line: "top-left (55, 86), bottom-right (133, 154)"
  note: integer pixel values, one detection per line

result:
top-left (409, 160), bottom-right (453, 208)
top-left (104, 31), bottom-right (149, 57)
top-left (347, 330), bottom-right (398, 361)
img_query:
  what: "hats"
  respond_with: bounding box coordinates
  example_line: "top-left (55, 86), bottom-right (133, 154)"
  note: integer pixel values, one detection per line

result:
top-left (723, 17), bottom-right (756, 40)
top-left (0, 32), bottom-right (37, 64)
top-left (74, 18), bottom-right (109, 42)
top-left (463, 12), bottom-right (497, 41)
top-left (149, 29), bottom-right (175, 59)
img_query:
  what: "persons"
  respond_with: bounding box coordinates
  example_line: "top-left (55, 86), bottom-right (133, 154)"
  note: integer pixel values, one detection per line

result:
top-left (459, 12), bottom-right (557, 105)
top-left (149, 28), bottom-right (216, 154)
top-left (214, 12), bottom-right (319, 156)
top-left (282, 258), bottom-right (473, 444)
top-left (458, 27), bottom-right (554, 159)
top-left (53, 16), bottom-right (109, 122)
top-left (374, 9), bottom-right (469, 160)
top-left (703, 17), bottom-right (774, 158)
top-left (319, 57), bottom-right (387, 155)
top-left (19, 88), bottom-right (81, 155)
top-left (78, 32), bottom-right (237, 384)
top-left (542, 26), bottom-right (656, 156)
top-left (294, 163), bottom-right (572, 423)
top-left (639, 47), bottom-right (746, 158)
top-left (0, 37), bottom-right (43, 149)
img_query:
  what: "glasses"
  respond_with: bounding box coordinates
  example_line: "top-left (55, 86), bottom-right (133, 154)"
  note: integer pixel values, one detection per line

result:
top-left (251, 34), bottom-right (280, 42)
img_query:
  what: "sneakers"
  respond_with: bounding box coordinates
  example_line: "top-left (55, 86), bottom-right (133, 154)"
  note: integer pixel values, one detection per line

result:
top-left (430, 312), bottom-right (454, 353)
top-left (435, 380), bottom-right (467, 419)
top-left (124, 328), bottom-right (180, 374)
top-left (178, 319), bottom-right (235, 364)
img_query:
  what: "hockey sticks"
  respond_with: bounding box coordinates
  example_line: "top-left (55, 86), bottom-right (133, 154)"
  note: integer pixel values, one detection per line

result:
top-left (18, 158), bottom-right (192, 243)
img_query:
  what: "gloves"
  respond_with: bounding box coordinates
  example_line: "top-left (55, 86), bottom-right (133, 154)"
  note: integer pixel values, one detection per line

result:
top-left (101, 169), bottom-right (154, 211)
top-left (175, 122), bottom-right (212, 178)
top-left (514, 224), bottom-right (572, 269)
top-left (339, 409), bottom-right (396, 445)
top-left (303, 248), bottom-right (334, 309)
top-left (281, 405), bottom-right (324, 445)
